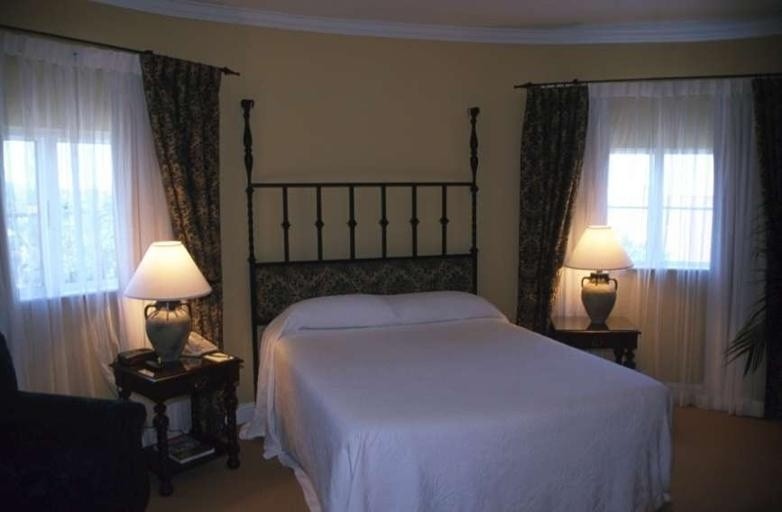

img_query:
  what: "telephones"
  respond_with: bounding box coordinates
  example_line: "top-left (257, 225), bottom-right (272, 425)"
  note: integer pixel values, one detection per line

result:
top-left (182, 331), bottom-right (217, 357)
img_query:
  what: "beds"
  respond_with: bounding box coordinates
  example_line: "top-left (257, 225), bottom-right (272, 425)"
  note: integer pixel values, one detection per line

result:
top-left (238, 97), bottom-right (675, 512)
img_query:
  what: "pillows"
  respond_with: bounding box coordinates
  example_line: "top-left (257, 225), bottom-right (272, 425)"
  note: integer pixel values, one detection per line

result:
top-left (286, 293), bottom-right (399, 329)
top-left (383, 288), bottom-right (500, 325)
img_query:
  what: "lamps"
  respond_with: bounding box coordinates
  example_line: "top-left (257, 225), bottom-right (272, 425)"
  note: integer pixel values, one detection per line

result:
top-left (563, 224), bottom-right (634, 329)
top-left (123, 239), bottom-right (213, 363)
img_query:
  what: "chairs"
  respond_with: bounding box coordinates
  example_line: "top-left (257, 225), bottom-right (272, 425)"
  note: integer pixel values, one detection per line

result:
top-left (0, 332), bottom-right (149, 512)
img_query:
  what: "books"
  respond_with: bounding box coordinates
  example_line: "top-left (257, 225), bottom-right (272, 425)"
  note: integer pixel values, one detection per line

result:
top-left (154, 433), bottom-right (216, 463)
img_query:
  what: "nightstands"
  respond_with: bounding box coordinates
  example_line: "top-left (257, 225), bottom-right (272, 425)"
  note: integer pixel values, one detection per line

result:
top-left (549, 313), bottom-right (642, 369)
top-left (108, 349), bottom-right (244, 497)
